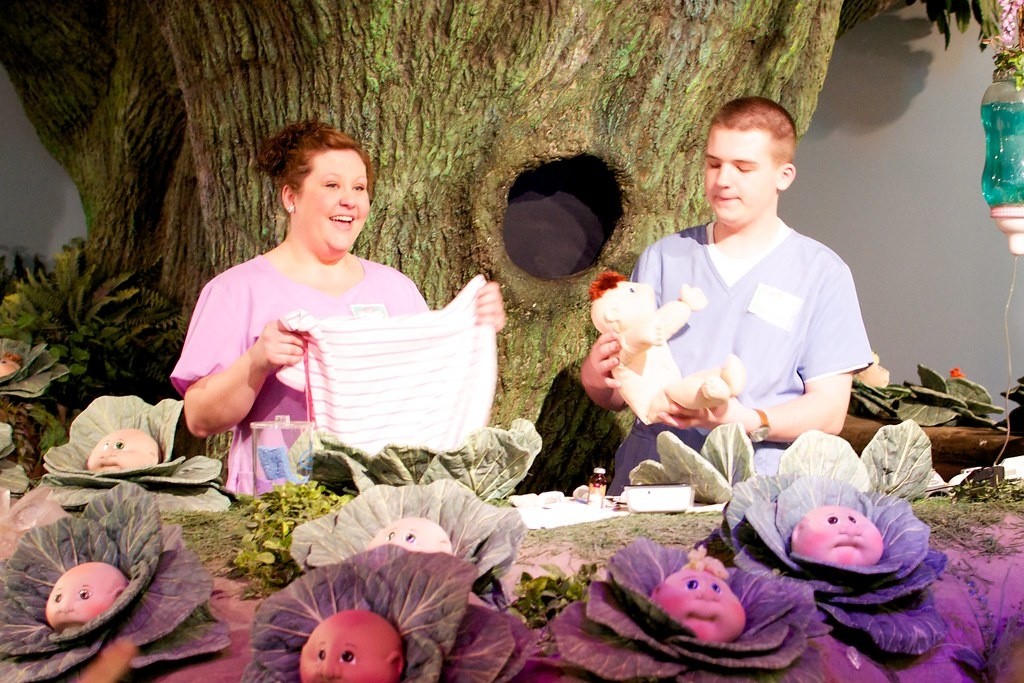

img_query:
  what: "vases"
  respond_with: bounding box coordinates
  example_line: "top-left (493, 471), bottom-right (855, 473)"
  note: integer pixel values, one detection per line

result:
top-left (979, 80), bottom-right (1024, 257)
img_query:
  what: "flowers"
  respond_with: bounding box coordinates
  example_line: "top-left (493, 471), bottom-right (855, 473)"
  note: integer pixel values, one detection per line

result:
top-left (982, 1), bottom-right (1024, 90)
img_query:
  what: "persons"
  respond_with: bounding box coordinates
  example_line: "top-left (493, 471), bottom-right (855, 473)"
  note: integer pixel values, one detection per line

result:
top-left (300, 610), bottom-right (404, 683)
top-left (652, 546), bottom-right (745, 643)
top-left (87, 429), bottom-right (162, 472)
top-left (46, 563), bottom-right (129, 632)
top-left (0, 353), bottom-right (23, 378)
top-left (856, 352), bottom-right (890, 389)
top-left (791, 505), bottom-right (884, 568)
top-left (581, 97), bottom-right (873, 498)
top-left (370, 517), bottom-right (453, 559)
top-left (169, 122), bottom-right (504, 499)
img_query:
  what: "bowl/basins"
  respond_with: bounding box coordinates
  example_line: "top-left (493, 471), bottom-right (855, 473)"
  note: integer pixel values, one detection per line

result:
top-left (624, 484), bottom-right (691, 513)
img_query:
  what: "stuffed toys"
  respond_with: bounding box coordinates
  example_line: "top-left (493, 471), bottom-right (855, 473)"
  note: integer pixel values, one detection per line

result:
top-left (591, 272), bottom-right (744, 425)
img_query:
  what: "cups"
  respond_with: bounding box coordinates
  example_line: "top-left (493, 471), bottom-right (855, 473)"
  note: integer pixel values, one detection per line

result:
top-left (251, 415), bottom-right (315, 501)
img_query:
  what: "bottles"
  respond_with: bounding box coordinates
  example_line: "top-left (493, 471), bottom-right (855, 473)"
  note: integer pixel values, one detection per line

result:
top-left (980, 80), bottom-right (1024, 255)
top-left (588, 468), bottom-right (608, 508)
